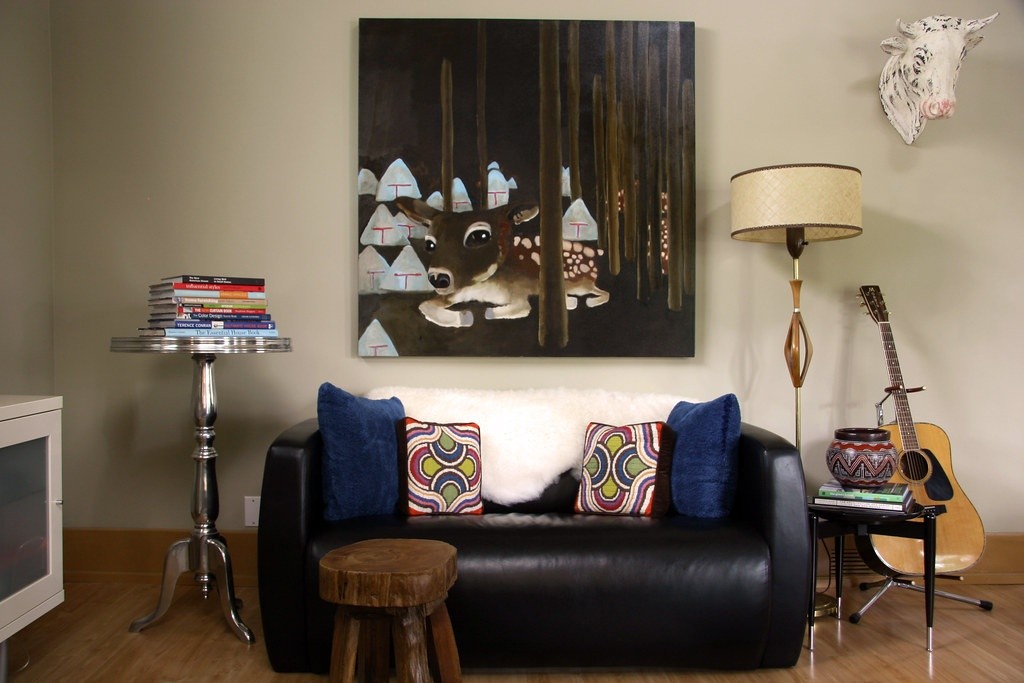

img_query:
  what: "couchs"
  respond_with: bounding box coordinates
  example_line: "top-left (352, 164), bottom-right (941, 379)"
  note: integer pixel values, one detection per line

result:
top-left (257, 390), bottom-right (806, 672)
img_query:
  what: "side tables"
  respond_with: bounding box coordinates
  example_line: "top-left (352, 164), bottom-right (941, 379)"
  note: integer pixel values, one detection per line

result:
top-left (809, 504), bottom-right (946, 652)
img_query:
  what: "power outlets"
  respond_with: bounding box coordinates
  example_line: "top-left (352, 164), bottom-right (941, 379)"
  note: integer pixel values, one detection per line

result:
top-left (245, 496), bottom-right (260, 527)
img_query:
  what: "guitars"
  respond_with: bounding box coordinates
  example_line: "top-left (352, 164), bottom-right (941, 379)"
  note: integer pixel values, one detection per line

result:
top-left (857, 285), bottom-right (987, 578)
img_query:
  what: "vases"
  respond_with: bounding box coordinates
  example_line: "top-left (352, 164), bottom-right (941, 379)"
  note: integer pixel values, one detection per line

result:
top-left (827, 427), bottom-right (896, 490)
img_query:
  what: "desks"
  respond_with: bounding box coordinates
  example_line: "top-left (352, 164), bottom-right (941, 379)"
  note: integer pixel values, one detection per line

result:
top-left (111, 335), bottom-right (293, 643)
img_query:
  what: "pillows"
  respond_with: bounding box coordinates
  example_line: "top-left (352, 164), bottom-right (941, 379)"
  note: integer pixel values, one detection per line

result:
top-left (573, 421), bottom-right (671, 516)
top-left (317, 380), bottom-right (405, 520)
top-left (664, 395), bottom-right (740, 516)
top-left (398, 416), bottom-right (482, 516)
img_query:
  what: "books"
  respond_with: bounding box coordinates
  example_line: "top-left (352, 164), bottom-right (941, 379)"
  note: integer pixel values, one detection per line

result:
top-left (812, 476), bottom-right (914, 512)
top-left (137, 275), bottom-right (278, 338)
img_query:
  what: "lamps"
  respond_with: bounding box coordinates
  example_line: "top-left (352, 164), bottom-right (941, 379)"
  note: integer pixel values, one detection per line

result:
top-left (732, 164), bottom-right (863, 620)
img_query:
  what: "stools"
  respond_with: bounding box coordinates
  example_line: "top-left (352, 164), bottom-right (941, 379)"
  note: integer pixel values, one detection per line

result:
top-left (320, 540), bottom-right (463, 683)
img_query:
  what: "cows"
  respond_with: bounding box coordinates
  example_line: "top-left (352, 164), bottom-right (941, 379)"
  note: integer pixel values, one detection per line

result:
top-left (877, 11), bottom-right (1002, 147)
